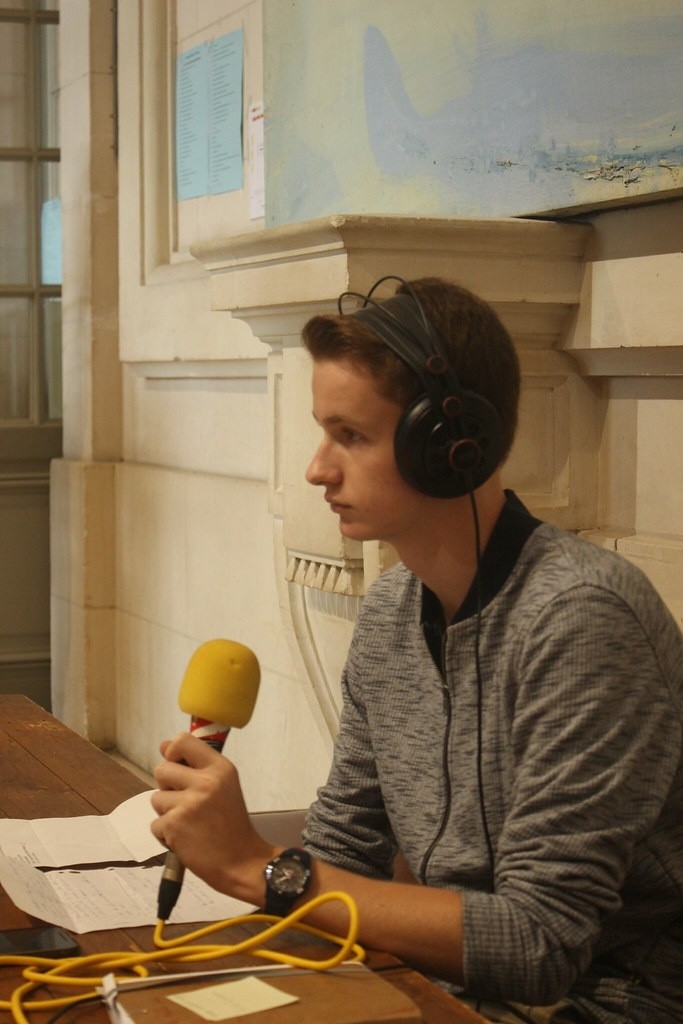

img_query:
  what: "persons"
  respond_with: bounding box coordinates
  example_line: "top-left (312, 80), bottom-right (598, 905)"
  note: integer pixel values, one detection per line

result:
top-left (151, 276), bottom-right (683, 1024)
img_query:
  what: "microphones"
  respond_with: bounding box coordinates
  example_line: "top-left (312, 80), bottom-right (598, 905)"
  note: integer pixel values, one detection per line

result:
top-left (156, 638), bottom-right (261, 920)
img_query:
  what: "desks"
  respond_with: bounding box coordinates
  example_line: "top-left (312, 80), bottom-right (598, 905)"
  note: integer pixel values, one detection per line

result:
top-left (0, 694), bottom-right (492, 1024)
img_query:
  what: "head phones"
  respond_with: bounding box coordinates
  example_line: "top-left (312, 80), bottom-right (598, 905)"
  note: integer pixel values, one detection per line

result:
top-left (338, 275), bottom-right (502, 498)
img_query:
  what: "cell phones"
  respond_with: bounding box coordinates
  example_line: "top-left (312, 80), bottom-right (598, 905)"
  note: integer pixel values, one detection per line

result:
top-left (0, 926), bottom-right (79, 958)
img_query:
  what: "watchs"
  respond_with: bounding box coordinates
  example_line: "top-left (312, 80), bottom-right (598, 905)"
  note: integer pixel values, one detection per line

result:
top-left (262, 847), bottom-right (314, 919)
